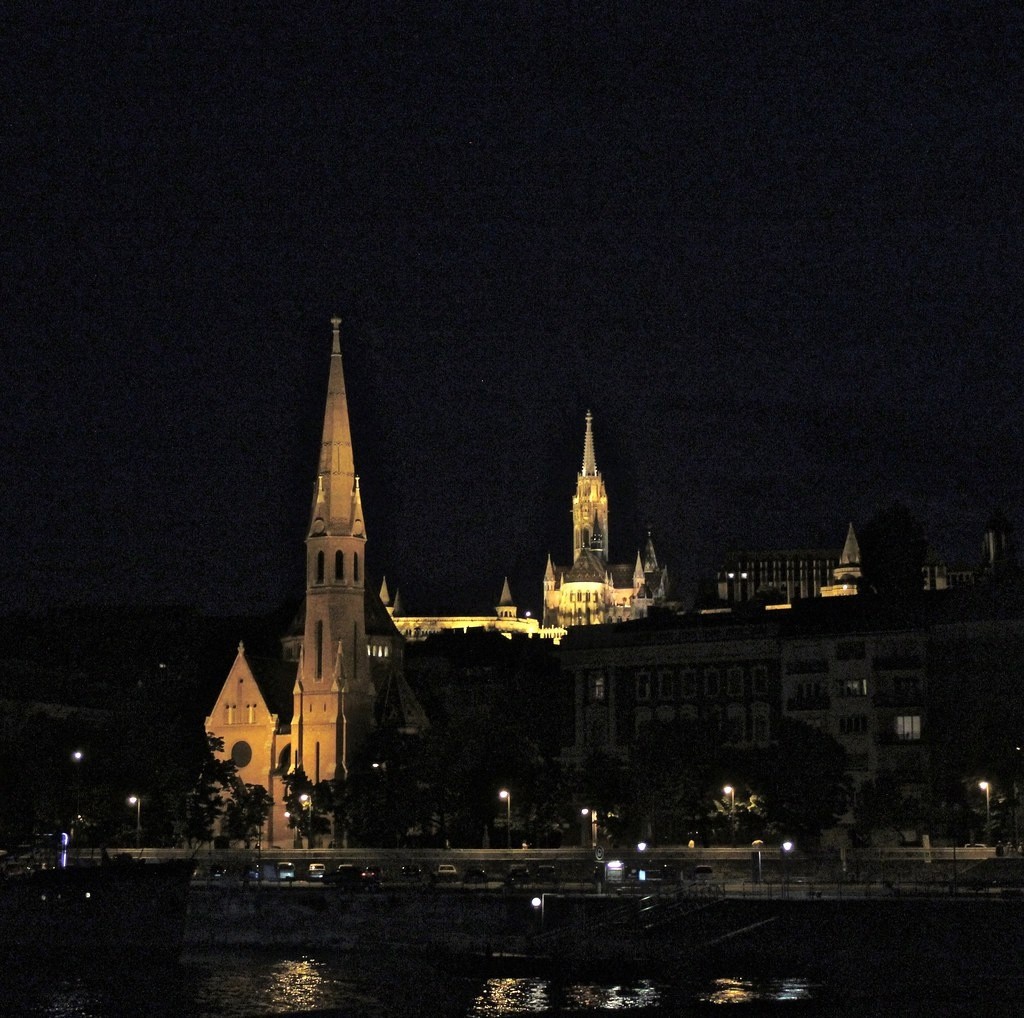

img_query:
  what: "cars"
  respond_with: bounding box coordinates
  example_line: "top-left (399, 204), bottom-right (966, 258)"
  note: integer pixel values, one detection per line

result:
top-left (210, 858), bottom-right (716, 886)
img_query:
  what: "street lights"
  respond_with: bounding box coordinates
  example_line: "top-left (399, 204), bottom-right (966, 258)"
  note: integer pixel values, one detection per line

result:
top-left (498, 789), bottom-right (511, 849)
top-left (783, 840), bottom-right (795, 899)
top-left (129, 794), bottom-right (140, 847)
top-left (71, 750), bottom-right (83, 848)
top-left (723, 785), bottom-right (737, 847)
top-left (979, 781), bottom-right (991, 824)
top-left (298, 793), bottom-right (312, 834)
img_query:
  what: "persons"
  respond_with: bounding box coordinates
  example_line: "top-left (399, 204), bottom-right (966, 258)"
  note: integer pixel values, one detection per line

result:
top-left (1005, 842), bottom-right (1024, 858)
top-left (995, 841), bottom-right (1004, 856)
